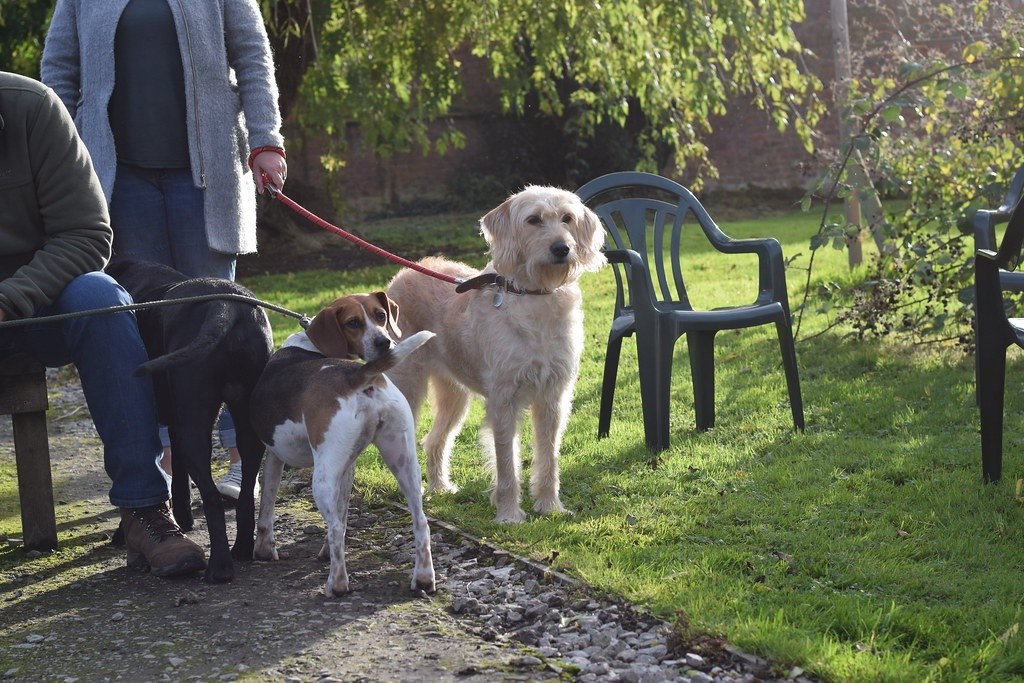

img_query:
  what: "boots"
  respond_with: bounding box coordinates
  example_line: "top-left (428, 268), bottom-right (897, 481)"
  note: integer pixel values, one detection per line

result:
top-left (121, 502), bottom-right (206, 579)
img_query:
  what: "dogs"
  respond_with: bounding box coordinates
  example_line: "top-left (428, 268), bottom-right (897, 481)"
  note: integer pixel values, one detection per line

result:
top-left (386, 185), bottom-right (609, 526)
top-left (249, 291), bottom-right (436, 598)
top-left (104, 258), bottom-right (274, 587)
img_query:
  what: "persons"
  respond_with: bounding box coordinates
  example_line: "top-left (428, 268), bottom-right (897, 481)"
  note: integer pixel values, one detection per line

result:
top-left (40, 0), bottom-right (287, 507)
top-left (0, 69), bottom-right (209, 576)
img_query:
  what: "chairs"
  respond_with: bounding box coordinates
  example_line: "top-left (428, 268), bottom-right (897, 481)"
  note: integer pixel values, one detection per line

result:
top-left (970, 157), bottom-right (1024, 488)
top-left (566, 171), bottom-right (807, 453)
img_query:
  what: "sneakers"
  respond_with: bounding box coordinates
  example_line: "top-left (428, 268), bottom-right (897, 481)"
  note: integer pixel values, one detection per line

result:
top-left (217, 464), bottom-right (260, 502)
